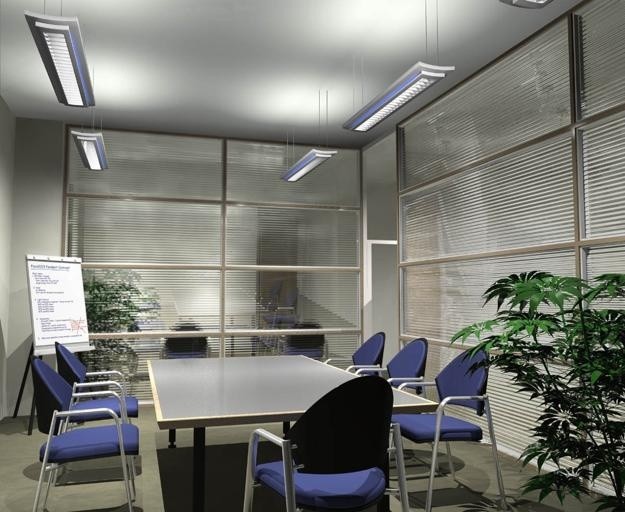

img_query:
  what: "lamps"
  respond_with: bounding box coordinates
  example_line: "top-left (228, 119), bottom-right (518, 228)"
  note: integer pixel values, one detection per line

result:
top-left (22, 0), bottom-right (99, 115)
top-left (67, 99), bottom-right (113, 178)
top-left (340, 0), bottom-right (461, 134)
top-left (501, 0), bottom-right (553, 12)
top-left (275, 79), bottom-right (340, 184)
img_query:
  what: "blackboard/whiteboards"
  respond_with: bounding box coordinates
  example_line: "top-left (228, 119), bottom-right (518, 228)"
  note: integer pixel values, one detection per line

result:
top-left (25, 254), bottom-right (96, 357)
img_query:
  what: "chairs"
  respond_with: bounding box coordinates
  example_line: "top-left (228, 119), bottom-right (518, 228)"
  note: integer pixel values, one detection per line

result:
top-left (160, 284), bottom-right (326, 359)
top-left (244, 374), bottom-right (394, 512)
top-left (29, 339), bottom-right (140, 512)
top-left (325, 333), bottom-right (386, 374)
top-left (387, 344), bottom-right (507, 512)
top-left (356, 337), bottom-right (429, 398)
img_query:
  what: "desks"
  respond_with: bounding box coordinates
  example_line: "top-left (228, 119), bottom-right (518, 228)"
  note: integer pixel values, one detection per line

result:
top-left (148, 355), bottom-right (439, 512)
